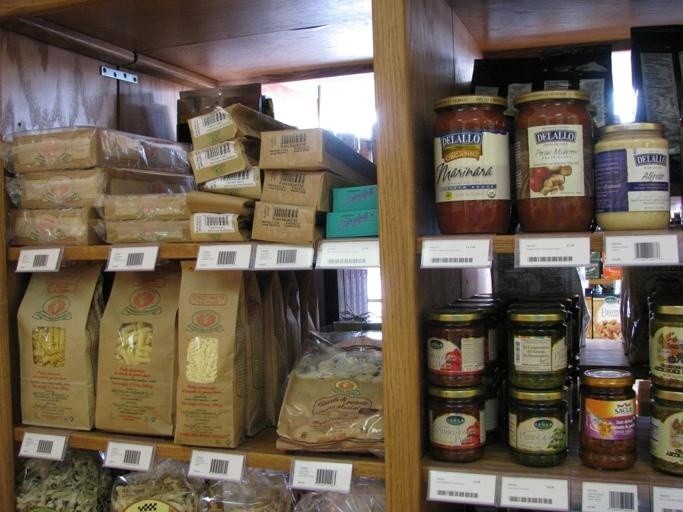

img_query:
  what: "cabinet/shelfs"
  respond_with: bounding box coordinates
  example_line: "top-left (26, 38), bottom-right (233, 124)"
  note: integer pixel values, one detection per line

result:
top-left (0, 1), bottom-right (682, 512)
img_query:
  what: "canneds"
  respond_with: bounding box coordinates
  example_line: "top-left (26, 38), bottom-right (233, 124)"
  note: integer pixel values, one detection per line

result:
top-left (594, 122), bottom-right (671, 233)
top-left (510, 90), bottom-right (596, 233)
top-left (579, 368), bottom-right (636, 472)
top-left (649, 387), bottom-right (683, 477)
top-left (648, 303), bottom-right (683, 391)
top-left (425, 292), bottom-right (581, 467)
top-left (432, 94), bottom-right (512, 235)
